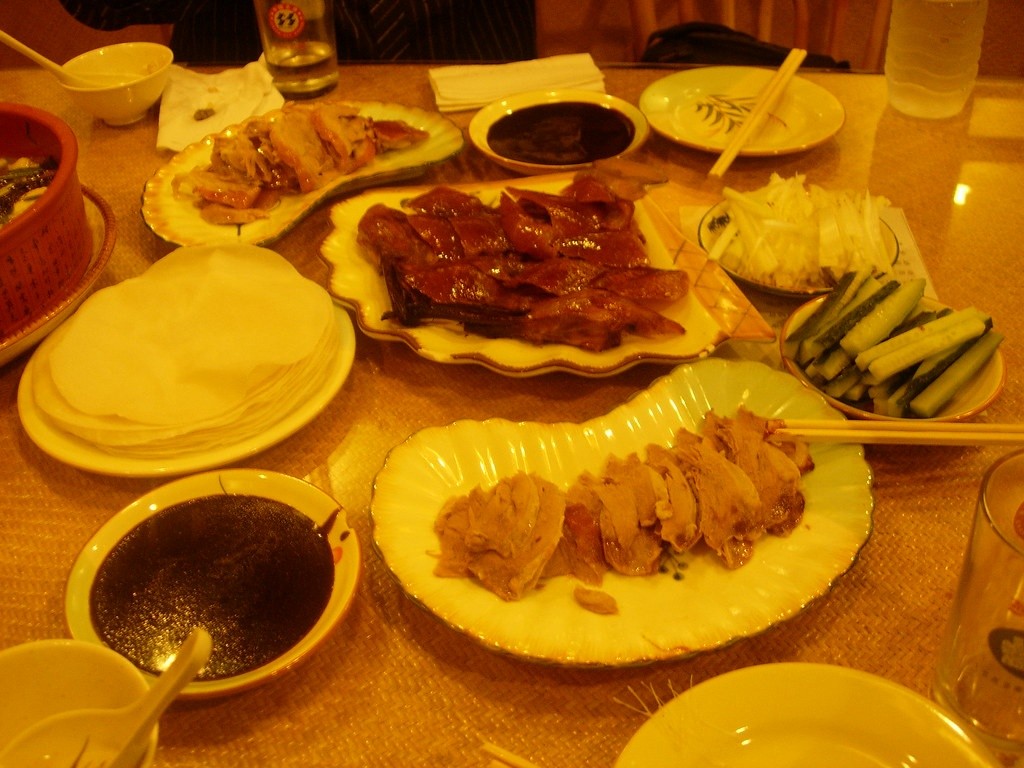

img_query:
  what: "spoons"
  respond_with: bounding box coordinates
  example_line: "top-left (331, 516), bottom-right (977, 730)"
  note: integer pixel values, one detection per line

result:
top-left (1, 626), bottom-right (213, 768)
top-left (0, 29), bottom-right (145, 88)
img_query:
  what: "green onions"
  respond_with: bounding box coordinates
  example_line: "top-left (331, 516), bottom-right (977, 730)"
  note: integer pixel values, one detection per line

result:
top-left (707, 169), bottom-right (895, 281)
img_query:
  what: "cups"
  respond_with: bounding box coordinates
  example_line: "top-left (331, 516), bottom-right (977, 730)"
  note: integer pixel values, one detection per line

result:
top-left (253, 0), bottom-right (340, 100)
top-left (924, 448), bottom-right (1024, 755)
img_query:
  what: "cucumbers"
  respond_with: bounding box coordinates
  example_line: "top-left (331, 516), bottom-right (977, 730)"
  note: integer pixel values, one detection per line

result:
top-left (782, 270), bottom-right (1005, 418)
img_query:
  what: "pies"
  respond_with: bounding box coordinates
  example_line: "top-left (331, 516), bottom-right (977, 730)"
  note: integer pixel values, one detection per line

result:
top-left (31, 242), bottom-right (342, 458)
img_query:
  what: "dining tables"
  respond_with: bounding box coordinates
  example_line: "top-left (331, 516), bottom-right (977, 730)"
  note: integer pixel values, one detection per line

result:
top-left (0, 62), bottom-right (1024, 768)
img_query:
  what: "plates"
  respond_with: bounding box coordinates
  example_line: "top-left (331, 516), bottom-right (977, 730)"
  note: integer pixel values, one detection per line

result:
top-left (639, 65), bottom-right (844, 157)
top-left (140, 102), bottom-right (470, 248)
top-left (13, 300), bottom-right (357, 477)
top-left (368, 358), bottom-right (876, 669)
top-left (469, 87), bottom-right (651, 174)
top-left (614, 661), bottom-right (1005, 767)
top-left (0, 180), bottom-right (118, 368)
top-left (62, 467), bottom-right (362, 699)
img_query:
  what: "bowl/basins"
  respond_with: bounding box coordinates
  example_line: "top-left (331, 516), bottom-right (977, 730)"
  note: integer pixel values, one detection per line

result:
top-left (57, 42), bottom-right (174, 126)
top-left (0, 101), bottom-right (97, 344)
top-left (779, 292), bottom-right (1008, 421)
top-left (698, 191), bottom-right (901, 297)
top-left (1, 640), bottom-right (161, 768)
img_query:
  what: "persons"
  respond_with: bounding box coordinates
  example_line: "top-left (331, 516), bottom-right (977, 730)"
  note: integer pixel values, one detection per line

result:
top-left (57, 0), bottom-right (538, 62)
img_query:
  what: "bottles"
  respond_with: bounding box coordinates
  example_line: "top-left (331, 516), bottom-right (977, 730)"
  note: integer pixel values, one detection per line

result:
top-left (885, 0), bottom-right (988, 120)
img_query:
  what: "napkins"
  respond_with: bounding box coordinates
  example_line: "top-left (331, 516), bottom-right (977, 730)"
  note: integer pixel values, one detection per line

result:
top-left (428, 52), bottom-right (607, 114)
top-left (156, 52), bottom-right (285, 153)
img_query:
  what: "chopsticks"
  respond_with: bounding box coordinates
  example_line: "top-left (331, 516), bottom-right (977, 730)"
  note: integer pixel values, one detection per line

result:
top-left (706, 48), bottom-right (806, 180)
top-left (772, 418), bottom-right (1024, 446)
